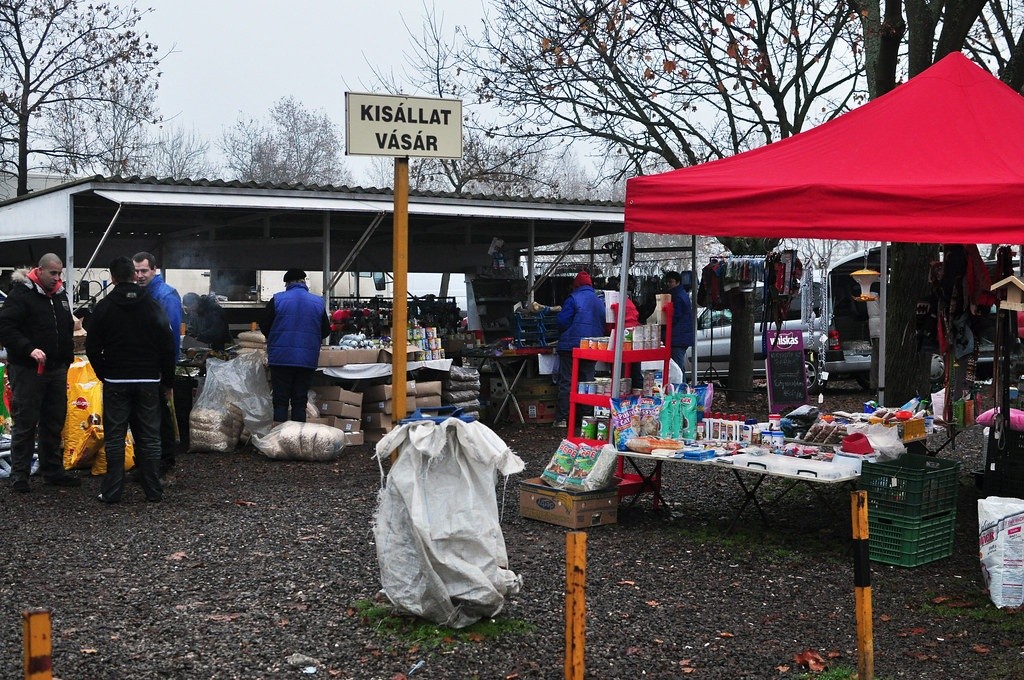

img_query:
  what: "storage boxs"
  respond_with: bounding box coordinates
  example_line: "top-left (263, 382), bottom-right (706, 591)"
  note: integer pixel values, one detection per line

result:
top-left (306, 340), bottom-right (557, 445)
top-left (860, 453), bottom-right (961, 567)
top-left (518, 477), bottom-right (619, 529)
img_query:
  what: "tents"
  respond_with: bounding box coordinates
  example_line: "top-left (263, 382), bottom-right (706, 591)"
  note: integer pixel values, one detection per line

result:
top-left (608, 52), bottom-right (1024, 413)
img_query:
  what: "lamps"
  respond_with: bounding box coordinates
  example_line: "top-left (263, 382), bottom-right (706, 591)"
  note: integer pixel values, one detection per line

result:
top-left (849, 241), bottom-right (881, 302)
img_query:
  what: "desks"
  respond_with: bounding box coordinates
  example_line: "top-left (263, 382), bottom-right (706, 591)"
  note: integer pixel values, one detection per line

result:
top-left (616, 434), bottom-right (941, 539)
top-left (453, 347), bottom-right (553, 426)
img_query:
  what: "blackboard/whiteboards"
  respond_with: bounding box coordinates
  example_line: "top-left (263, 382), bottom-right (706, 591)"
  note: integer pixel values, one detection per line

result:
top-left (767, 349), bottom-right (810, 410)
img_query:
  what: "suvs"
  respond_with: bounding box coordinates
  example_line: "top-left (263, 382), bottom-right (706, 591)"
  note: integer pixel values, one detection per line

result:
top-left (685, 244), bottom-right (912, 396)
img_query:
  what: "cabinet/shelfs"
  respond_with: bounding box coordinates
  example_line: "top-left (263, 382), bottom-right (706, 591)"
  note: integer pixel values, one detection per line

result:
top-left (464, 279), bottom-right (557, 343)
top-left (567, 344), bottom-right (672, 509)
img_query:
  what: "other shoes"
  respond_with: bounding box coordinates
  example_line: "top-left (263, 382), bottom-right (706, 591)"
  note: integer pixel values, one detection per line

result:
top-left (553, 419), bottom-right (567, 427)
top-left (125, 467), bottom-right (141, 482)
top-left (14, 481), bottom-right (30, 493)
top-left (98, 494), bottom-right (113, 504)
top-left (43, 474), bottom-right (80, 486)
top-left (158, 461), bottom-right (175, 476)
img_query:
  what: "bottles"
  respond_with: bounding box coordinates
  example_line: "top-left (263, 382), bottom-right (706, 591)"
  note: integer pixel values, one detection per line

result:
top-left (702, 411), bottom-right (759, 445)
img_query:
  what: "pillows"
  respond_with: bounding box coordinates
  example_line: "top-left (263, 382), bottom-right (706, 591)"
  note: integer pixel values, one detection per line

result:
top-left (976, 406), bottom-right (1024, 431)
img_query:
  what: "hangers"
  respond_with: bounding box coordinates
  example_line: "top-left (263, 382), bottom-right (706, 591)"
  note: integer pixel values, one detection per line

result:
top-left (328, 297), bottom-right (393, 313)
top-left (527, 252), bottom-right (794, 278)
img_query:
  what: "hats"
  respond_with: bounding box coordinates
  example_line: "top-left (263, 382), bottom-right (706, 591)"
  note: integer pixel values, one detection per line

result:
top-left (283, 268), bottom-right (307, 282)
top-left (574, 272), bottom-right (592, 288)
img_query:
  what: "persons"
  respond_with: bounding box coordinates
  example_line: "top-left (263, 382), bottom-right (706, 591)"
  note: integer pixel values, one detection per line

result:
top-left (182, 293), bottom-right (229, 351)
top-left (84, 255), bottom-right (177, 504)
top-left (0, 251), bottom-right (82, 491)
top-left (552, 270), bottom-right (606, 428)
top-left (603, 273), bottom-right (644, 388)
top-left (662, 269), bottom-right (694, 382)
top-left (258, 269), bottom-right (331, 428)
top-left (132, 252), bottom-right (183, 476)
top-left (329, 310), bottom-right (358, 346)
